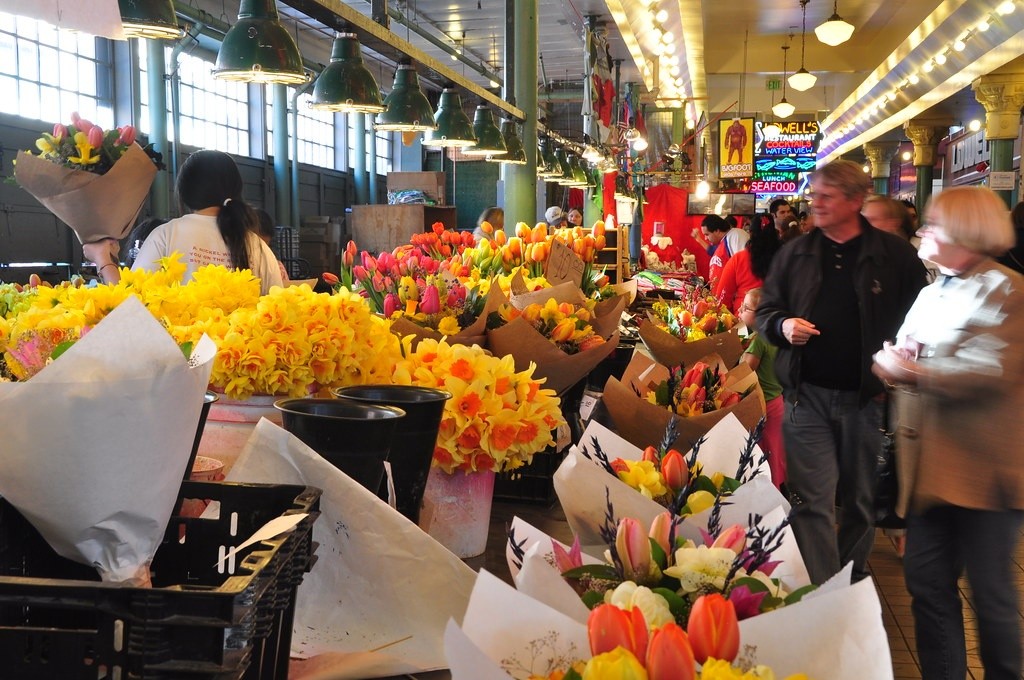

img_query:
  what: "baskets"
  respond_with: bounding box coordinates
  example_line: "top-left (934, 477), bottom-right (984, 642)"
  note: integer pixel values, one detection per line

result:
top-left (0, 479), bottom-right (323, 680)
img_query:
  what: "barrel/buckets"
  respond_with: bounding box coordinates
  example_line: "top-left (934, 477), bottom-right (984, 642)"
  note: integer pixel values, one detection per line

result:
top-left (272, 384), bottom-right (454, 524)
top-left (178, 455), bottom-right (226, 539)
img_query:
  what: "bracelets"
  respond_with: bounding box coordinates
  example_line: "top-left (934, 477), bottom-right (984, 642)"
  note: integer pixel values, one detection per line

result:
top-left (98, 263), bottom-right (118, 278)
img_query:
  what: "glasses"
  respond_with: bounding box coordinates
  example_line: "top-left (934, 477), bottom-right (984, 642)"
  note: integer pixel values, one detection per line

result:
top-left (740, 302), bottom-right (755, 313)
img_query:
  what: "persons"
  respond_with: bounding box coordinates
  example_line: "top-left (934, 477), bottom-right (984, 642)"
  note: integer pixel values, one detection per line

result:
top-left (545, 206), bottom-right (567, 228)
top-left (701, 199), bottom-right (1024, 559)
top-left (472, 207), bottom-right (504, 248)
top-left (248, 207), bottom-right (289, 286)
top-left (82, 150), bottom-right (284, 297)
top-left (568, 205), bottom-right (583, 226)
top-left (737, 287), bottom-right (789, 500)
top-left (753, 160), bottom-right (931, 587)
top-left (870, 185), bottom-right (1024, 680)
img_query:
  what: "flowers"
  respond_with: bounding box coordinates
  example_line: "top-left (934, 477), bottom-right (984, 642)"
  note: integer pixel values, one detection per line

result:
top-left (0, 220), bottom-right (819, 680)
top-left (12, 110), bottom-right (167, 177)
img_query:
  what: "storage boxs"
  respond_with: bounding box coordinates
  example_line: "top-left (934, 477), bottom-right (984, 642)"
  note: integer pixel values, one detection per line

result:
top-left (387, 172), bottom-right (445, 205)
top-left (0, 472), bottom-right (323, 680)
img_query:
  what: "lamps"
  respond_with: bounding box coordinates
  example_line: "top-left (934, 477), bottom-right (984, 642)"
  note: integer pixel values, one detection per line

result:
top-left (118, 0), bottom-right (181, 39)
top-left (211, 0), bottom-right (306, 84)
top-left (814, 0), bottom-right (855, 47)
top-left (306, 0), bottom-right (597, 189)
top-left (787, 0), bottom-right (818, 92)
top-left (771, 46), bottom-right (796, 118)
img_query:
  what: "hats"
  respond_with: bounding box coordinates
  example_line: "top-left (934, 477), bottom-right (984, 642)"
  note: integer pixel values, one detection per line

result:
top-left (544, 206), bottom-right (564, 225)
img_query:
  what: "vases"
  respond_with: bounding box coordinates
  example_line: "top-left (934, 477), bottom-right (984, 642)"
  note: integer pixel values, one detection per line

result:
top-left (272, 397), bottom-right (408, 497)
top-left (193, 395), bottom-right (296, 481)
top-left (418, 456), bottom-right (496, 560)
top-left (331, 384), bottom-right (452, 527)
